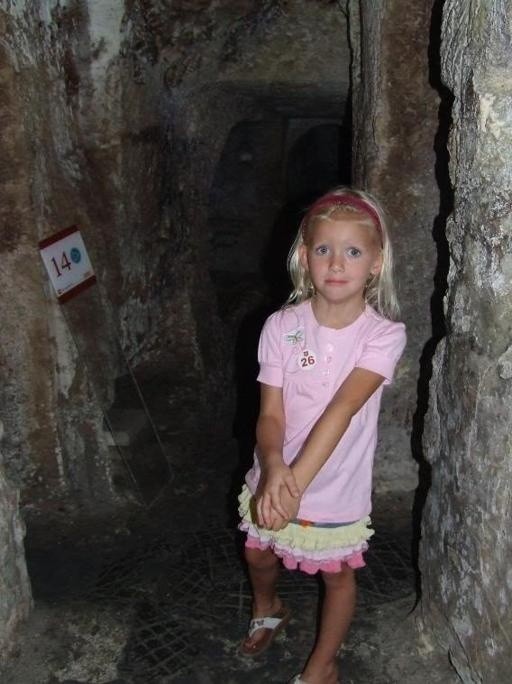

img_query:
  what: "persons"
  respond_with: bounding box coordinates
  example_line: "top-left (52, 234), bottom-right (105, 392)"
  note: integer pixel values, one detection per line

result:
top-left (237, 185), bottom-right (407, 684)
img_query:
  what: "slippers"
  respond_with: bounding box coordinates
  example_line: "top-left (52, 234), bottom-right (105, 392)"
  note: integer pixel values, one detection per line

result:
top-left (240, 606), bottom-right (293, 657)
